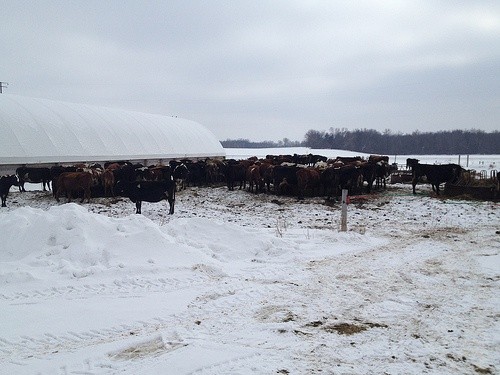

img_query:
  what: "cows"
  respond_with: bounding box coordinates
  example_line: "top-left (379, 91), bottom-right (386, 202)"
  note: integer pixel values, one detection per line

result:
top-left (412, 163), bottom-right (465, 196)
top-left (117, 180), bottom-right (176, 215)
top-left (406, 158), bottom-right (421, 170)
top-left (0, 153), bottom-right (398, 207)
